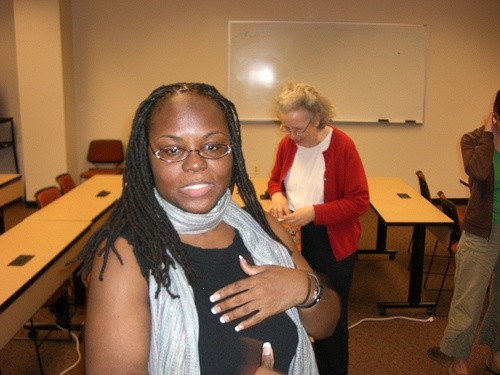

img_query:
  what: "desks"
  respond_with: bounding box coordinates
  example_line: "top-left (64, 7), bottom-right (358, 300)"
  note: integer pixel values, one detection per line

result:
top-left (0, 173), bottom-right (24, 233)
top-left (0, 217), bottom-right (93, 375)
top-left (353, 176), bottom-right (455, 316)
top-left (227, 173), bottom-right (281, 213)
top-left (26, 174), bottom-right (130, 332)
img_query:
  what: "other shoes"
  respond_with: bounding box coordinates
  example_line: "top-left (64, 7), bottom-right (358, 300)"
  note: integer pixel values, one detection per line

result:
top-left (486, 356), bottom-right (500, 375)
top-left (448, 363), bottom-right (469, 375)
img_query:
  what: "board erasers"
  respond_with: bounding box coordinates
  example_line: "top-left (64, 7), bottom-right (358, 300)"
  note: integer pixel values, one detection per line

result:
top-left (405, 119), bottom-right (416, 123)
top-left (378, 119), bottom-right (389, 122)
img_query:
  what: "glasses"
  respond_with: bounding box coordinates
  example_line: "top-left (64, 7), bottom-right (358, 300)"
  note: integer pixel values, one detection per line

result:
top-left (146, 143), bottom-right (231, 161)
top-left (280, 118), bottom-right (313, 133)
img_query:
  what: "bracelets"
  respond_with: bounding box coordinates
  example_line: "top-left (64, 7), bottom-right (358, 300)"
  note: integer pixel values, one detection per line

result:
top-left (296, 272), bottom-right (323, 308)
top-left (300, 277), bottom-right (311, 305)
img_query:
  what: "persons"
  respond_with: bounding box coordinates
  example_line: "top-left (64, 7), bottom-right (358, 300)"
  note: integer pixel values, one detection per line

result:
top-left (77, 82), bottom-right (342, 375)
top-left (267, 82), bottom-right (369, 375)
top-left (439, 90), bottom-right (500, 375)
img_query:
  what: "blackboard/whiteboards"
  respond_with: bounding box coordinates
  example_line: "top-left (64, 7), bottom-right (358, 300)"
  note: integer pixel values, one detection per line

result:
top-left (227, 20), bottom-right (426, 124)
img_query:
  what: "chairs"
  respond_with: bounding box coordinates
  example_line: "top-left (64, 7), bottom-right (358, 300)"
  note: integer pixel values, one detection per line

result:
top-left (34, 139), bottom-right (131, 208)
top-left (407, 171), bottom-right (462, 320)
